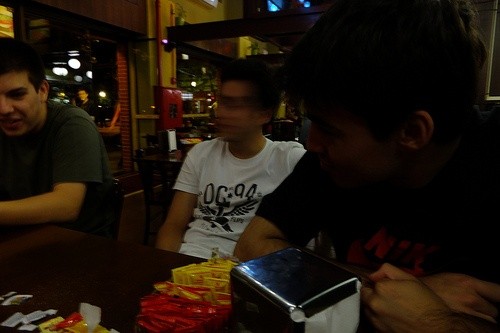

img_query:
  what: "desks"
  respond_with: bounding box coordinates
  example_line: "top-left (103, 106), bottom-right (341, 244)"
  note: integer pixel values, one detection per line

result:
top-left (0, 225), bottom-right (208, 333)
top-left (136, 147), bottom-right (193, 231)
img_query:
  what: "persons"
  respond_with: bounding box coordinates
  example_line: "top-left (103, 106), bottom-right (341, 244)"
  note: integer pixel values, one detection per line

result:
top-left (72, 90), bottom-right (97, 121)
top-left (231, 0), bottom-right (500, 333)
top-left (157, 59), bottom-right (308, 259)
top-left (0, 37), bottom-right (114, 235)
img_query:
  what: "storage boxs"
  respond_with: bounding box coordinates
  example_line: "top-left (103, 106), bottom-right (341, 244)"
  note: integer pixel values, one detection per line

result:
top-left (229, 247), bottom-right (362, 333)
